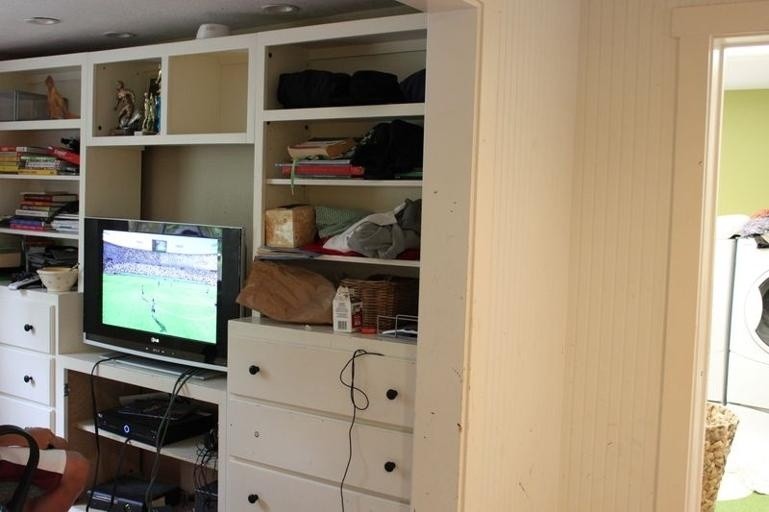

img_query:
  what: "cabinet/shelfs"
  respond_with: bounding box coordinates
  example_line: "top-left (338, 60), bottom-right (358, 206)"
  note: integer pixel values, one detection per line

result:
top-left (0, 11), bottom-right (428, 512)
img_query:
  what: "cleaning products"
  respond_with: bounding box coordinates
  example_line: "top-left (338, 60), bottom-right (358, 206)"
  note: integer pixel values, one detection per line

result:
top-left (332, 285), bottom-right (353, 332)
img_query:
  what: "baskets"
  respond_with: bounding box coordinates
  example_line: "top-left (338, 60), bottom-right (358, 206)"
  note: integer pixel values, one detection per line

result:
top-left (338, 277), bottom-right (419, 330)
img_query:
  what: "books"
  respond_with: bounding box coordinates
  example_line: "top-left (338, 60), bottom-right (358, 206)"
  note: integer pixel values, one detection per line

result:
top-left (0, 137), bottom-right (79, 236)
top-left (272, 134), bottom-right (366, 181)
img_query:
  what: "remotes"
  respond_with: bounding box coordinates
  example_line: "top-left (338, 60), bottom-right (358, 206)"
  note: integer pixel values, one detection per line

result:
top-left (8, 276), bottom-right (41, 289)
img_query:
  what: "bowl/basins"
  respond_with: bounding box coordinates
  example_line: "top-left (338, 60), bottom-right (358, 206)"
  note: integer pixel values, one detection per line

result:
top-left (36, 266), bottom-right (78, 293)
top-left (196, 24), bottom-right (231, 39)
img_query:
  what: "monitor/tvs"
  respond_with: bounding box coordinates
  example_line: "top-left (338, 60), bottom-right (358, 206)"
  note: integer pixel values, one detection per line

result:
top-left (83, 217), bottom-right (247, 381)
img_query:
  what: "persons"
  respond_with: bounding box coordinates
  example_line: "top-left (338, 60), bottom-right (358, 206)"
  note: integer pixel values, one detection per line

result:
top-left (142, 285), bottom-right (144, 298)
top-left (102, 238), bottom-right (217, 287)
top-left (151, 299), bottom-right (156, 316)
top-left (45, 75), bottom-right (67, 118)
top-left (109, 62), bottom-right (162, 137)
